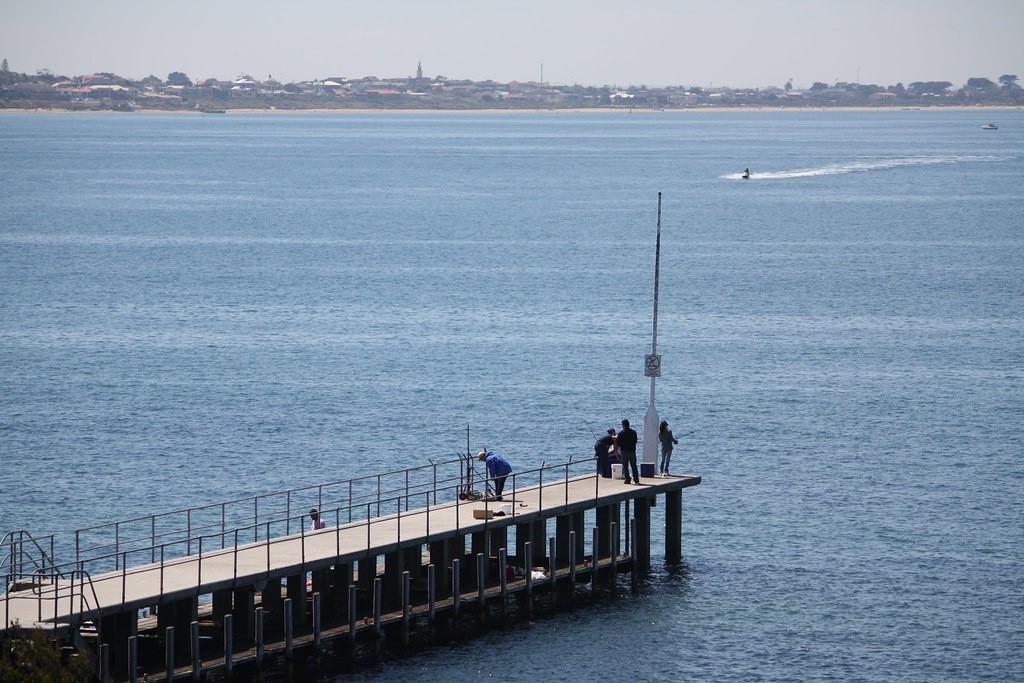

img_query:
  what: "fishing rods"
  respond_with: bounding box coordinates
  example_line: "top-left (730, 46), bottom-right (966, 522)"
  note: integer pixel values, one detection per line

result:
top-left (676, 421), bottom-right (717, 440)
top-left (590, 430), bottom-right (598, 441)
top-left (446, 442), bottom-right (496, 492)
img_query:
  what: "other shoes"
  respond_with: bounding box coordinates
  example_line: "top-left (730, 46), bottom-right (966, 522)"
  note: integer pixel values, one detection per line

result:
top-left (495, 496), bottom-right (503, 501)
top-left (624, 479), bottom-right (631, 484)
top-left (659, 472), bottom-right (663, 476)
top-left (634, 480), bottom-right (640, 484)
top-left (664, 473), bottom-right (671, 475)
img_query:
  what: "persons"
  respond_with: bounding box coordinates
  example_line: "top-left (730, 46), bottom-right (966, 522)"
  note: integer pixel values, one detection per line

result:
top-left (310, 510), bottom-right (325, 530)
top-left (617, 419), bottom-right (639, 485)
top-left (658, 421), bottom-right (674, 476)
top-left (594, 428), bottom-right (618, 477)
top-left (478, 451), bottom-right (512, 501)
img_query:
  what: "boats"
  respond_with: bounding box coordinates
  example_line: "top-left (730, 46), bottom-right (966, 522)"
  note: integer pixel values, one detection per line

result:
top-left (981, 125), bottom-right (998, 130)
top-left (741, 172), bottom-right (749, 178)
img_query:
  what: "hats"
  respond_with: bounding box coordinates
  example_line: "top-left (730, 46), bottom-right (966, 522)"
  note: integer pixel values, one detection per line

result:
top-left (606, 428), bottom-right (616, 434)
top-left (477, 451), bottom-right (486, 462)
top-left (659, 421), bottom-right (668, 425)
